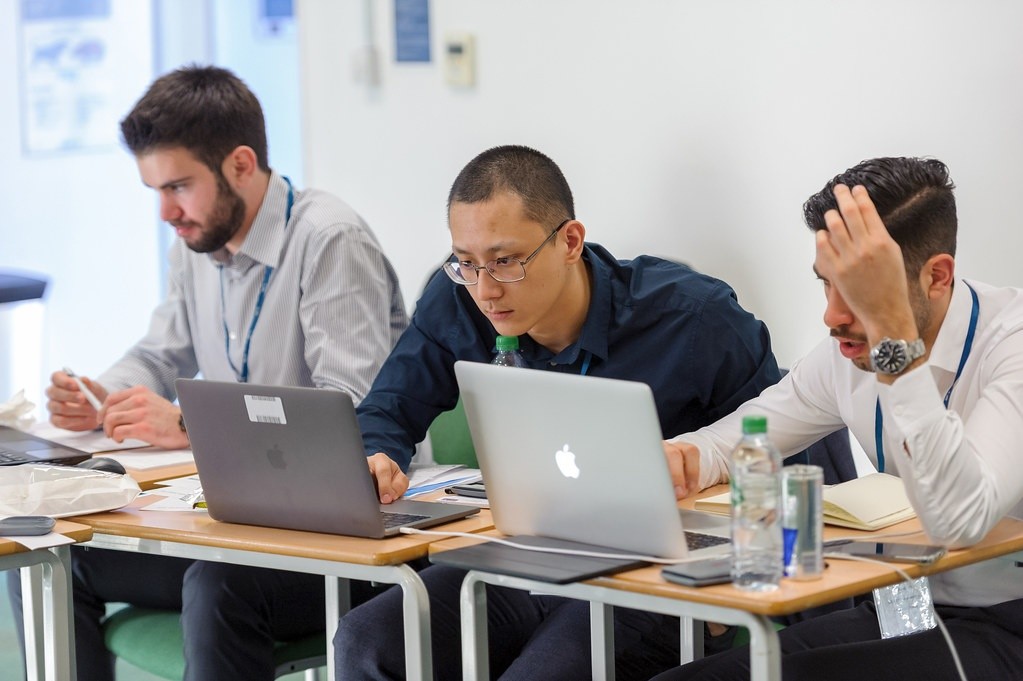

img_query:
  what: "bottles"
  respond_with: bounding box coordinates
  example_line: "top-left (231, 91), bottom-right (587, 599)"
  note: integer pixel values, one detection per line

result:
top-left (489, 335), bottom-right (531, 368)
top-left (727, 412), bottom-right (785, 595)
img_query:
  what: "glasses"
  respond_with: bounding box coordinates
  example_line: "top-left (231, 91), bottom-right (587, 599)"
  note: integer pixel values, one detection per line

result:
top-left (442, 220), bottom-right (568, 285)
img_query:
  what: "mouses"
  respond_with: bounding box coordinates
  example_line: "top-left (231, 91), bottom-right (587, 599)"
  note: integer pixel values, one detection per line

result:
top-left (75, 457), bottom-right (126, 475)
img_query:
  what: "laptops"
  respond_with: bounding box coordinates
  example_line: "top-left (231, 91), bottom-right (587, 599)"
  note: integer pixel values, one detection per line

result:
top-left (175, 379), bottom-right (481, 540)
top-left (454, 360), bottom-right (733, 565)
top-left (0, 424), bottom-right (92, 467)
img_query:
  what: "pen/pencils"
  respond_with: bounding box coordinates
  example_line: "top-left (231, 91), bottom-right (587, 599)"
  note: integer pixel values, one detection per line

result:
top-left (822, 538), bottom-right (853, 548)
top-left (63, 367), bottom-right (104, 412)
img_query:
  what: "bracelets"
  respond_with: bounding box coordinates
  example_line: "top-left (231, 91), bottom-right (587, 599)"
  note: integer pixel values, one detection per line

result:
top-left (178, 414), bottom-right (188, 437)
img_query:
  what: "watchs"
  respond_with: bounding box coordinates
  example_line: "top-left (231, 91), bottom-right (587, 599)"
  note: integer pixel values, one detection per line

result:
top-left (870, 337), bottom-right (926, 377)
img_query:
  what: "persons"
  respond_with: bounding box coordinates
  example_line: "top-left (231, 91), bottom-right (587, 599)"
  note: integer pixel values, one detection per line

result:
top-left (5, 67), bottom-right (410, 681)
top-left (649, 156), bottom-right (1023, 681)
top-left (334, 146), bottom-right (783, 681)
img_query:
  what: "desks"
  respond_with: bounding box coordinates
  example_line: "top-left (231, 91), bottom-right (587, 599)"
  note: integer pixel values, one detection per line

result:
top-left (0, 449), bottom-right (1023, 681)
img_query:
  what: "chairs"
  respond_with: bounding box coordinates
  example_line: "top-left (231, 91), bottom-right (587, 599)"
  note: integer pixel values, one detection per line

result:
top-left (97, 378), bottom-right (482, 681)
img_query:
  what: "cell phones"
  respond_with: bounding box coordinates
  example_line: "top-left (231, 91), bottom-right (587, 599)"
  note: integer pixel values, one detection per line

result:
top-left (821, 539), bottom-right (946, 565)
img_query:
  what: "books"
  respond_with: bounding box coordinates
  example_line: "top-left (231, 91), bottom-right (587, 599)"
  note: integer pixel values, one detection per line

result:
top-left (90, 452), bottom-right (198, 484)
top-left (696, 471), bottom-right (918, 533)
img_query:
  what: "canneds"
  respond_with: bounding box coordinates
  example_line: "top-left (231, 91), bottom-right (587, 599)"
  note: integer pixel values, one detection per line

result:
top-left (779, 464), bottom-right (823, 582)
